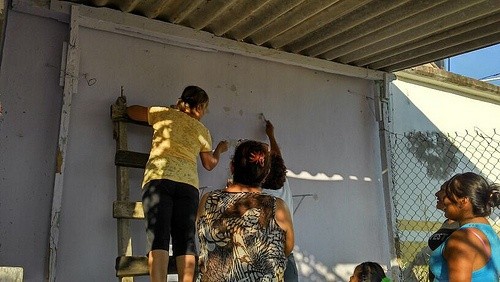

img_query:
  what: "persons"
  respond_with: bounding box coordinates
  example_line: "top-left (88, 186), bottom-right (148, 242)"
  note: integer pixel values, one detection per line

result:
top-left (428, 172), bottom-right (500, 282)
top-left (124, 86), bottom-right (228, 282)
top-left (349, 262), bottom-right (387, 282)
top-left (195, 139), bottom-right (295, 282)
top-left (428, 180), bottom-right (462, 282)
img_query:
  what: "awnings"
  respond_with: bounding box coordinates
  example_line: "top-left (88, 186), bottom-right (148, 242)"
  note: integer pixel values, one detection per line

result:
top-left (81, 0), bottom-right (500, 74)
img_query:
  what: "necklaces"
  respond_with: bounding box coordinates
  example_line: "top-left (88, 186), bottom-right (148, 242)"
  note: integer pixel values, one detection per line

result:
top-left (260, 120), bottom-right (298, 282)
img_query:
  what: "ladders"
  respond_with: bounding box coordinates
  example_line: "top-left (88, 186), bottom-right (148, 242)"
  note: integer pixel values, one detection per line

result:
top-left (110, 85), bottom-right (200, 282)
